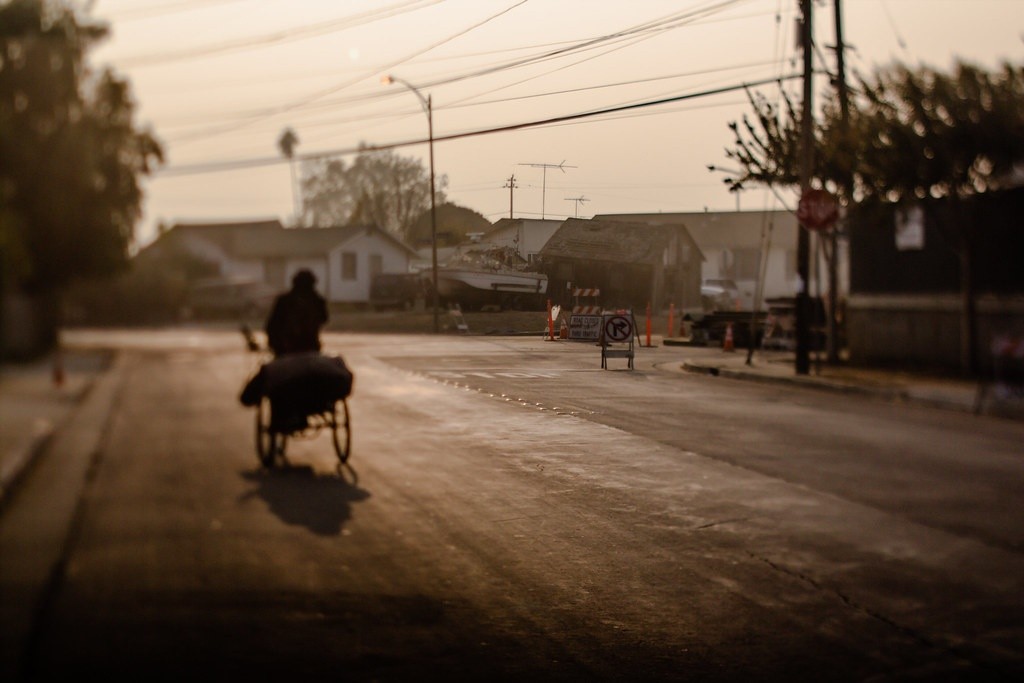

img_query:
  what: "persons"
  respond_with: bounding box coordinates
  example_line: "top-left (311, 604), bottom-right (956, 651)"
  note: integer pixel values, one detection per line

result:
top-left (262, 270), bottom-right (329, 356)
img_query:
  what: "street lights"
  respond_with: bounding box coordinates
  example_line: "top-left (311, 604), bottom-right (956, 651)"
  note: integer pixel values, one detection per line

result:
top-left (382, 76), bottom-right (438, 334)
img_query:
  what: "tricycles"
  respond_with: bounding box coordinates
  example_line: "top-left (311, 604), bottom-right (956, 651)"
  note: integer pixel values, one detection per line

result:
top-left (253, 355), bottom-right (353, 471)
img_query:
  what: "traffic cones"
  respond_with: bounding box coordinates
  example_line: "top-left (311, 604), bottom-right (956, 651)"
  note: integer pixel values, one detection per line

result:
top-left (556, 316), bottom-right (569, 340)
top-left (722, 322), bottom-right (736, 353)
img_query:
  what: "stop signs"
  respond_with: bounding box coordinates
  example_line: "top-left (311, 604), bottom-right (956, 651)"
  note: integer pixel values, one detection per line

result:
top-left (796, 189), bottom-right (838, 231)
